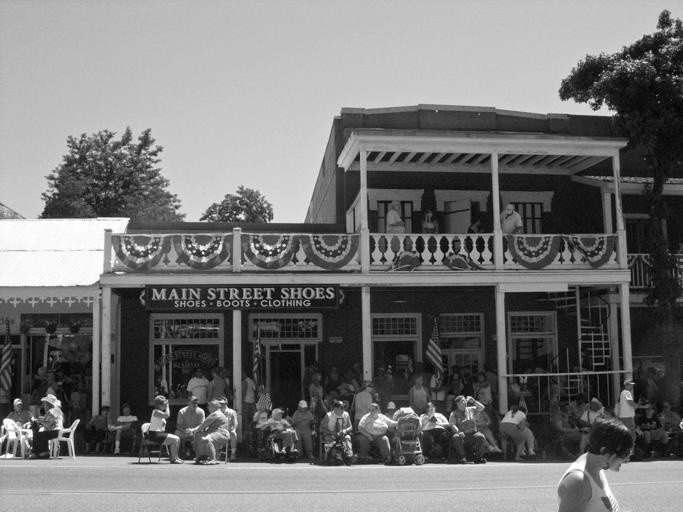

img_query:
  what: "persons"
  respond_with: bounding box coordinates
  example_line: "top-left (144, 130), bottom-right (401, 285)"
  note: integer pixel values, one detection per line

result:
top-left (419, 209), bottom-right (440, 234)
top-left (466, 218), bottom-right (480, 234)
top-left (541, 377), bottom-right (682, 459)
top-left (183, 364), bottom-right (234, 410)
top-left (217, 396), bottom-right (239, 462)
top-left (5, 397), bottom-right (33, 431)
top-left (174, 396), bottom-right (205, 459)
top-left (192, 400), bottom-right (230, 466)
top-left (146, 395), bottom-right (185, 465)
top-left (384, 199), bottom-right (405, 232)
top-left (83, 405), bottom-right (112, 455)
top-left (554, 418), bottom-right (633, 512)
top-left (241, 357), bottom-right (537, 467)
top-left (30, 372), bottom-right (86, 460)
top-left (108, 402), bottom-right (138, 457)
top-left (499, 202), bottom-right (523, 232)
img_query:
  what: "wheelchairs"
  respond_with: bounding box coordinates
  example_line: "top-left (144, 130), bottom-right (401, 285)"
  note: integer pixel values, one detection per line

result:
top-left (262, 426), bottom-right (298, 463)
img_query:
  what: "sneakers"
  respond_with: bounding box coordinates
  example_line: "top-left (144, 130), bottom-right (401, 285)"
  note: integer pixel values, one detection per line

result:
top-left (281, 450), bottom-right (286, 455)
top-left (488, 446), bottom-right (494, 452)
top-left (335, 458), bottom-right (343, 465)
top-left (290, 449), bottom-right (298, 455)
top-left (385, 459), bottom-right (392, 463)
top-left (204, 460), bottom-right (216, 465)
top-left (530, 451), bottom-right (535, 456)
top-left (458, 459), bottom-right (466, 463)
top-left (16, 453), bottom-right (22, 457)
top-left (364, 458), bottom-right (371, 464)
top-left (171, 458), bottom-right (184, 464)
top-left (475, 458), bottom-right (487, 463)
top-left (344, 456), bottom-right (352, 465)
top-left (519, 453), bottom-right (526, 457)
top-left (114, 448), bottom-right (120, 454)
top-left (33, 453), bottom-right (49, 458)
top-left (495, 447), bottom-right (502, 453)
top-left (307, 453), bottom-right (315, 460)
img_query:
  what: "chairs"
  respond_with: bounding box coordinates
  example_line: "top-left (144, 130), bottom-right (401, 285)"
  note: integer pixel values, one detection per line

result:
top-left (48, 417), bottom-right (82, 458)
top-left (3, 417), bottom-right (31, 459)
top-left (138, 421), bottom-right (164, 463)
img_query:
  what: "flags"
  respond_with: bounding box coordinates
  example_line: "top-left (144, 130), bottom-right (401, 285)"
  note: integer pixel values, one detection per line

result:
top-left (0, 320), bottom-right (17, 394)
top-left (424, 319), bottom-right (444, 390)
top-left (251, 321), bottom-right (263, 385)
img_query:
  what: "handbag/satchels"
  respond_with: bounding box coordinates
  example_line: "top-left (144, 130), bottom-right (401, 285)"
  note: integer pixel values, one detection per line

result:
top-left (458, 419), bottom-right (477, 435)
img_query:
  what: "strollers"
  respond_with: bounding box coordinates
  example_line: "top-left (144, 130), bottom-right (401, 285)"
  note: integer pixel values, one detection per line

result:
top-left (389, 406), bottom-right (424, 465)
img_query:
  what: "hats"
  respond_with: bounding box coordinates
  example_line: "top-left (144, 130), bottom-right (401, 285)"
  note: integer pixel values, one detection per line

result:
top-left (41, 394), bottom-right (57, 405)
top-left (14, 399), bottom-right (22, 405)
top-left (387, 401), bottom-right (396, 409)
top-left (624, 380), bottom-right (635, 385)
top-left (299, 400), bottom-right (308, 408)
top-left (272, 408), bottom-right (284, 415)
